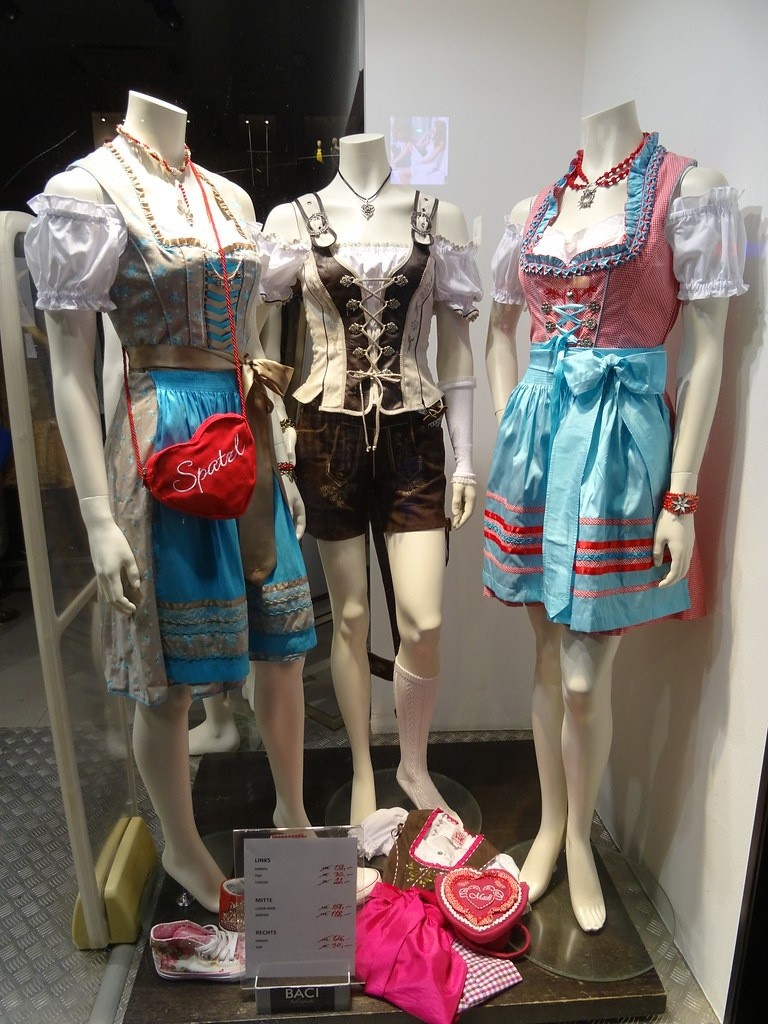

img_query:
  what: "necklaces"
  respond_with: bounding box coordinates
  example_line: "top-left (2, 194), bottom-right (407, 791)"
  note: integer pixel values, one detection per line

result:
top-left (116, 123), bottom-right (195, 227)
top-left (338, 166), bottom-right (392, 221)
top-left (567, 132), bottom-right (651, 210)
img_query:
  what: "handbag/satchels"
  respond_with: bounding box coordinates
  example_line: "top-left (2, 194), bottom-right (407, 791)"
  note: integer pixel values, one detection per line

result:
top-left (141, 414), bottom-right (257, 520)
top-left (435, 865), bottom-right (531, 959)
top-left (352, 881), bottom-right (468, 1024)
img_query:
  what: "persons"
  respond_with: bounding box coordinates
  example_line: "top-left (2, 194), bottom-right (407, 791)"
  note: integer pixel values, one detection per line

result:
top-left (24, 91), bottom-right (320, 908)
top-left (481, 97), bottom-right (749, 933)
top-left (260, 134), bottom-right (482, 848)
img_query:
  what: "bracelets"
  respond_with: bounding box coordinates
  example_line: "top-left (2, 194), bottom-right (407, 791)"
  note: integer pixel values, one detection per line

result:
top-left (662, 490), bottom-right (699, 515)
top-left (277, 461), bottom-right (298, 482)
top-left (280, 418), bottom-right (297, 433)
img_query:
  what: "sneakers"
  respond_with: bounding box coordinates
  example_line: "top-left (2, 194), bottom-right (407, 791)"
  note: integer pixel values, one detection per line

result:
top-left (218, 866), bottom-right (382, 933)
top-left (150, 920), bottom-right (247, 982)
